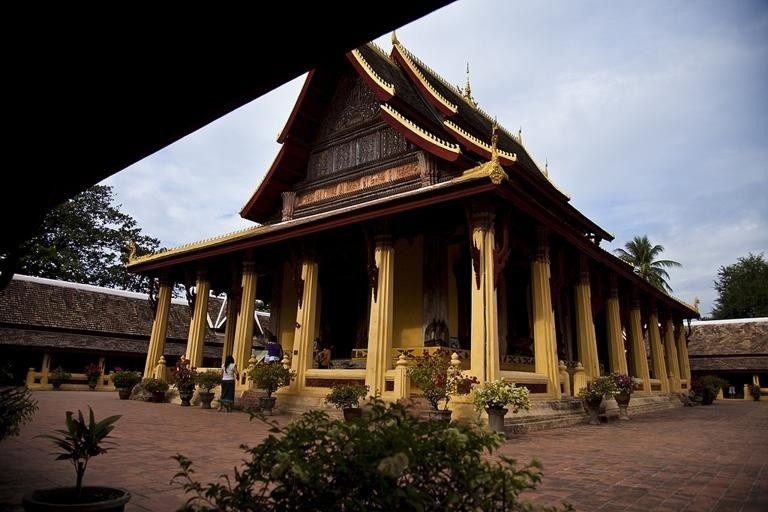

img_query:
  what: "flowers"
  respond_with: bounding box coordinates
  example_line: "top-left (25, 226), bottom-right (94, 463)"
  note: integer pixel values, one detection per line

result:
top-left (110, 367), bottom-right (141, 390)
top-left (471, 374), bottom-right (530, 417)
top-left (172, 357), bottom-right (198, 391)
top-left (611, 372), bottom-right (640, 394)
top-left (395, 345), bottom-right (481, 410)
top-left (577, 375), bottom-right (620, 401)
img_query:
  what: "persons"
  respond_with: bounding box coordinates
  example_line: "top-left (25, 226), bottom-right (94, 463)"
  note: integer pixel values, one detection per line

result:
top-left (217, 355), bottom-right (239, 412)
top-left (264, 336), bottom-right (283, 364)
top-left (319, 343), bottom-right (334, 369)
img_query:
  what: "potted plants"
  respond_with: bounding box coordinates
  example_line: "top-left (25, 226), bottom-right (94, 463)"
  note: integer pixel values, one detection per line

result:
top-left (325, 381), bottom-right (370, 420)
top-left (691, 375), bottom-right (724, 406)
top-left (747, 384), bottom-right (762, 401)
top-left (22, 403), bottom-right (131, 512)
top-left (140, 377), bottom-right (169, 403)
top-left (47, 366), bottom-right (72, 391)
top-left (247, 357), bottom-right (298, 415)
top-left (84, 363), bottom-right (102, 391)
top-left (195, 368), bottom-right (222, 409)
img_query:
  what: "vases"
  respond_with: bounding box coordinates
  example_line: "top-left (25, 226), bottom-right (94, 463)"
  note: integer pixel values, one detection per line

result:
top-left (613, 394), bottom-right (630, 421)
top-left (428, 410), bottom-right (453, 424)
top-left (119, 391), bottom-right (131, 399)
top-left (484, 408), bottom-right (507, 432)
top-left (180, 391), bottom-right (194, 406)
top-left (583, 397), bottom-right (602, 413)
